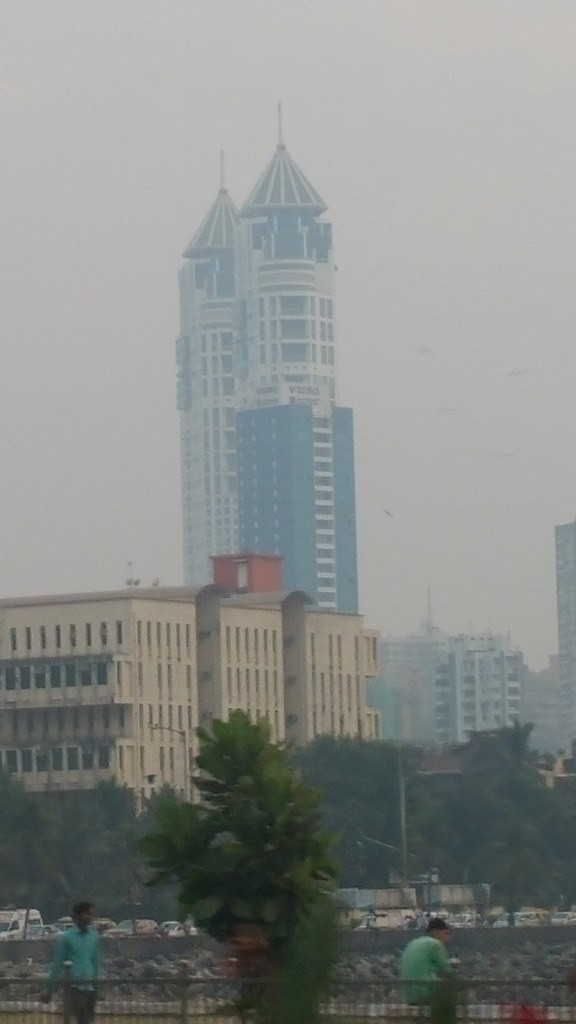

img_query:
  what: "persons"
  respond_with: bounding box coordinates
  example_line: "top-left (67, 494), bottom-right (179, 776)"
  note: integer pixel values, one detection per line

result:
top-left (397, 918), bottom-right (466, 1024)
top-left (38, 902), bottom-right (107, 1024)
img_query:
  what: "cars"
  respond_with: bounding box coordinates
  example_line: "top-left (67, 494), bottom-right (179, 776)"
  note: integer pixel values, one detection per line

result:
top-left (0, 881), bottom-right (575, 948)
